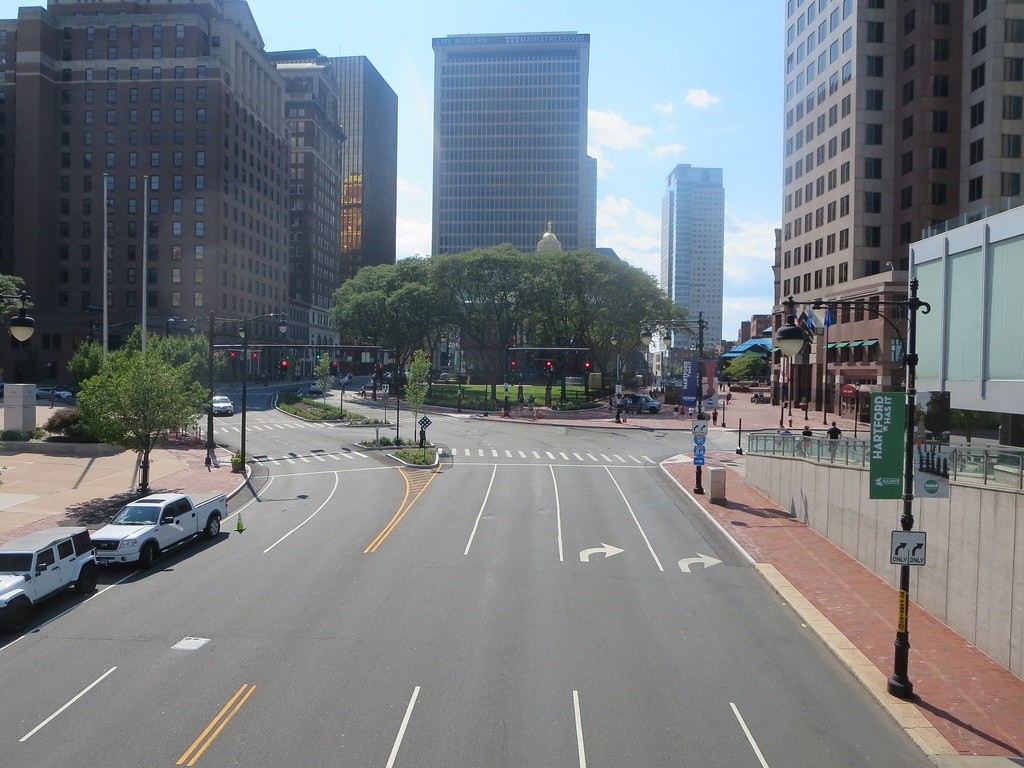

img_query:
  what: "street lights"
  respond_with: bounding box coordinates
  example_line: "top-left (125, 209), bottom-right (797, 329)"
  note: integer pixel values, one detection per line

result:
top-left (166, 317), bottom-right (199, 342)
top-left (240, 311), bottom-right (289, 471)
top-left (777, 277), bottom-right (931, 698)
top-left (207, 310), bottom-right (245, 448)
top-left (638, 310), bottom-right (710, 495)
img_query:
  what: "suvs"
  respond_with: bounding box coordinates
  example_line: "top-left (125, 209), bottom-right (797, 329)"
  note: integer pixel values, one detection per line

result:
top-left (0, 526), bottom-right (100, 636)
top-left (625, 394), bottom-right (661, 414)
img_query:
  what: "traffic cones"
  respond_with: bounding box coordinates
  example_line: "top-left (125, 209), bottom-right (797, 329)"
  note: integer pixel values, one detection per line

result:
top-left (234, 512), bottom-right (246, 530)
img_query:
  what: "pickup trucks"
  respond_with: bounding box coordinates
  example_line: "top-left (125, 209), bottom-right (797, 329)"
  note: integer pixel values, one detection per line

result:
top-left (90, 491), bottom-right (228, 569)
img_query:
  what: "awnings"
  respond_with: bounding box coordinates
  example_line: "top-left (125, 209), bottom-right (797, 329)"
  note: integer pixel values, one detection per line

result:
top-left (770, 348), bottom-right (779, 353)
top-left (823, 340), bottom-right (878, 348)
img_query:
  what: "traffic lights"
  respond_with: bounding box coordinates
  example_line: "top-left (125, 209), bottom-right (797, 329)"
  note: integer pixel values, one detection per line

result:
top-left (331, 359), bottom-right (338, 371)
top-left (316, 351), bottom-right (321, 359)
top-left (280, 354), bottom-right (288, 371)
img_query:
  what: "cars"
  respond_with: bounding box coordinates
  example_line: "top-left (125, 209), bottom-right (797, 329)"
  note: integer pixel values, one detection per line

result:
top-left (752, 392), bottom-right (766, 403)
top-left (310, 381), bottom-right (323, 393)
top-left (211, 395), bottom-right (234, 417)
top-left (361, 371), bottom-right (411, 390)
top-left (339, 372), bottom-right (354, 386)
top-left (36, 385), bottom-right (81, 400)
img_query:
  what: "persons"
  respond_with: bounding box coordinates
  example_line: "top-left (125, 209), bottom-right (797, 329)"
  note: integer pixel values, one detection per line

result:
top-left (49, 390), bottom-right (55, 408)
top-left (800, 425), bottom-right (813, 457)
top-left (674, 403), bottom-right (694, 422)
top-left (754, 393), bottom-right (764, 404)
top-left (826, 422), bottom-right (842, 457)
top-left (712, 408), bottom-right (718, 426)
top-left (726, 392), bottom-right (731, 405)
top-left (649, 389), bottom-right (657, 397)
top-left (775, 427), bottom-right (791, 450)
